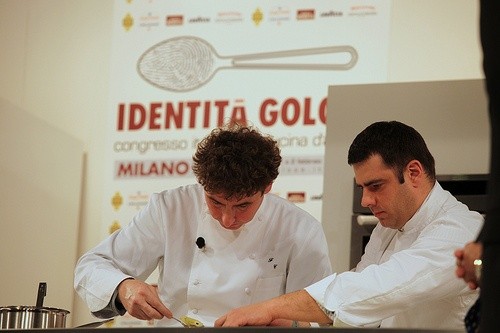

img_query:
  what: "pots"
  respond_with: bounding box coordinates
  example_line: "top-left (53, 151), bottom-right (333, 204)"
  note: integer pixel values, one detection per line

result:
top-left (0, 282), bottom-right (70, 330)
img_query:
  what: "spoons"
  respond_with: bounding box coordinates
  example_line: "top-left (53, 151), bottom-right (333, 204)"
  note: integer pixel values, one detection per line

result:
top-left (136, 35), bottom-right (359, 92)
top-left (171, 314), bottom-right (202, 332)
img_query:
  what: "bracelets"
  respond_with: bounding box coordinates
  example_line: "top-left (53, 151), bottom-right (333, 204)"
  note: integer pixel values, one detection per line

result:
top-left (293, 319), bottom-right (298, 328)
top-left (473, 253), bottom-right (484, 286)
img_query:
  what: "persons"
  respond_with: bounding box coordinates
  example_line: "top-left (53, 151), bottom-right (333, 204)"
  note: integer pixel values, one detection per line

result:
top-left (454, 0), bottom-right (500, 333)
top-left (215, 121), bottom-right (484, 333)
top-left (74, 118), bottom-right (334, 328)
